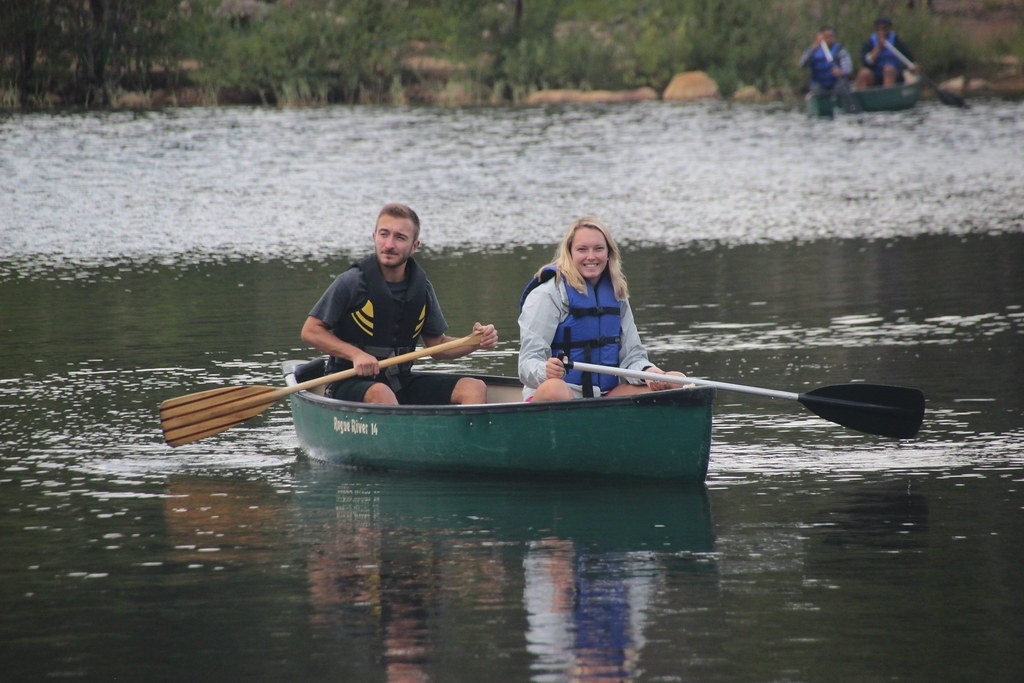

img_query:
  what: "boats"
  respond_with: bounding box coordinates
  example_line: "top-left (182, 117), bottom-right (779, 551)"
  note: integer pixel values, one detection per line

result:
top-left (808, 81), bottom-right (923, 119)
top-left (277, 354), bottom-right (717, 483)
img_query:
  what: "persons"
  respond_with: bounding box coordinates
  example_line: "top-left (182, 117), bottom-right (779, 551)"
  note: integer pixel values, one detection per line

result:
top-left (855, 17), bottom-right (919, 90)
top-left (800, 24), bottom-right (852, 94)
top-left (300, 203), bottom-right (498, 406)
top-left (517, 217), bottom-right (687, 403)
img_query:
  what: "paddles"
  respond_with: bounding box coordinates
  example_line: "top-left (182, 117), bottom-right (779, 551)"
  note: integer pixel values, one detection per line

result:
top-left (821, 39), bottom-right (862, 115)
top-left (884, 39), bottom-right (970, 109)
top-left (554, 349), bottom-right (925, 439)
top-left (158, 324), bottom-right (490, 448)
